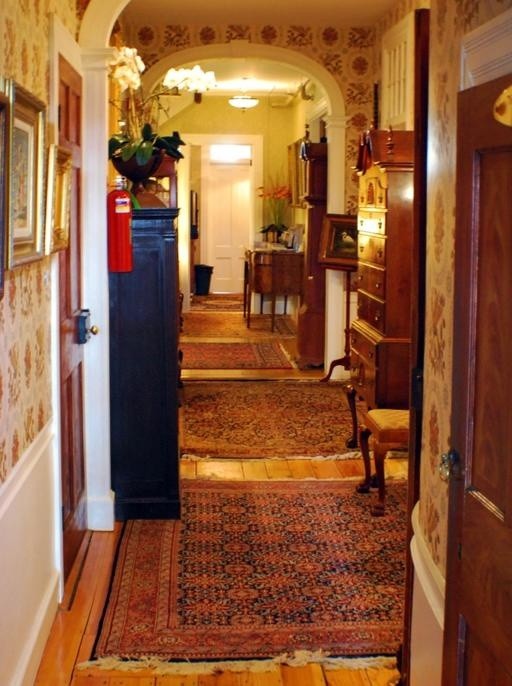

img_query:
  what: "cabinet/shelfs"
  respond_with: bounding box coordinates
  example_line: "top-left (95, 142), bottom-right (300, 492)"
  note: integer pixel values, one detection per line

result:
top-left (291, 141), bottom-right (327, 368)
top-left (108, 209), bottom-right (189, 525)
top-left (342, 117), bottom-right (416, 448)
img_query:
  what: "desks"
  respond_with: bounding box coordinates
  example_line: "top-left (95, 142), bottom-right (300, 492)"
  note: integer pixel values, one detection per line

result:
top-left (250, 247), bottom-right (305, 333)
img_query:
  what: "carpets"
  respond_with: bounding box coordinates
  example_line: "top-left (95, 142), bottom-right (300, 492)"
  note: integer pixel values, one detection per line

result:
top-left (81, 474), bottom-right (407, 675)
top-left (175, 340), bottom-right (297, 373)
top-left (176, 377), bottom-right (380, 461)
top-left (189, 292), bottom-right (246, 310)
top-left (180, 309), bottom-right (295, 340)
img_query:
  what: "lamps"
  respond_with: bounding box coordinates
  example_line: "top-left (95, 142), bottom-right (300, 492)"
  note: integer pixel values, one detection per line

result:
top-left (229, 95), bottom-right (260, 112)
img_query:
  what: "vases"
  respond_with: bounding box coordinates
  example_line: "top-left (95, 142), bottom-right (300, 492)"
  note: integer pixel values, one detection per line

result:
top-left (266, 228), bottom-right (282, 244)
top-left (113, 153), bottom-right (175, 208)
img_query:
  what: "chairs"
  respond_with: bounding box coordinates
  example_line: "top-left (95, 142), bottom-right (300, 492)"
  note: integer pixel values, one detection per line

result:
top-left (240, 252), bottom-right (276, 327)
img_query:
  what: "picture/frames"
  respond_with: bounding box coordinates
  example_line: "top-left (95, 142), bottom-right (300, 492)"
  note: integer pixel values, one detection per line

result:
top-left (3, 75), bottom-right (47, 270)
top-left (189, 189), bottom-right (199, 227)
top-left (317, 213), bottom-right (358, 266)
top-left (43, 142), bottom-right (74, 257)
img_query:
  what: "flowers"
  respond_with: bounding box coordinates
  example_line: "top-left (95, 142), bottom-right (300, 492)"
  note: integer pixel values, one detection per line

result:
top-left (106, 45), bottom-right (219, 165)
top-left (258, 172), bottom-right (293, 231)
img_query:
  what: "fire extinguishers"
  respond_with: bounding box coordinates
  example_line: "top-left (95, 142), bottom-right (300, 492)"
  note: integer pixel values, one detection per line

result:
top-left (107, 175), bottom-right (134, 273)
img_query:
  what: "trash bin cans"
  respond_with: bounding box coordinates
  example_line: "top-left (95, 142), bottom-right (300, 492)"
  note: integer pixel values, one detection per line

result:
top-left (195, 264), bottom-right (214, 295)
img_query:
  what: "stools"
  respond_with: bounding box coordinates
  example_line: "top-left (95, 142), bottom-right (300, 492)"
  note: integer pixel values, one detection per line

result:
top-left (353, 407), bottom-right (411, 516)
